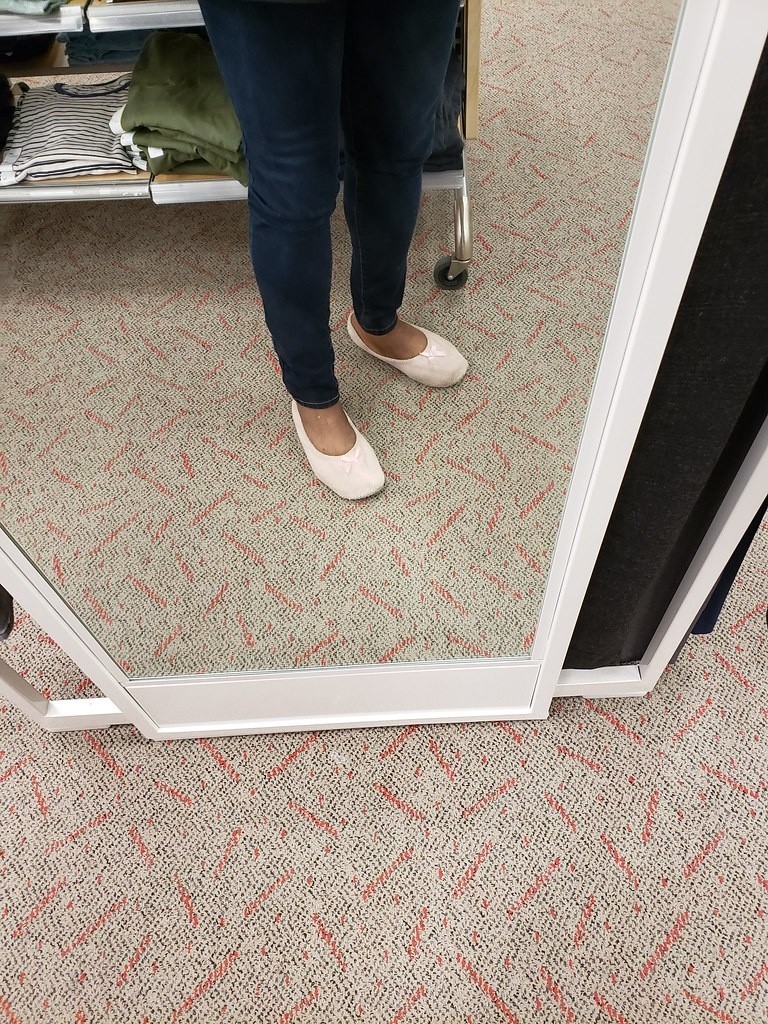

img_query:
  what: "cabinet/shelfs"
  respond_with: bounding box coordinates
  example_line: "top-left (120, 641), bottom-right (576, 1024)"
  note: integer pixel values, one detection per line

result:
top-left (0, 0), bottom-right (479, 289)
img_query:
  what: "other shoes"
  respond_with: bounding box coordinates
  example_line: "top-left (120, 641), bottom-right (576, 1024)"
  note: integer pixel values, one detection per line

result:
top-left (347, 311), bottom-right (468, 388)
top-left (291, 398), bottom-right (385, 499)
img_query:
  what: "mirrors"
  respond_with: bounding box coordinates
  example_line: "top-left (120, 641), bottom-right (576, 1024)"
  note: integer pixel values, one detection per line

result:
top-left (0, 0), bottom-right (768, 741)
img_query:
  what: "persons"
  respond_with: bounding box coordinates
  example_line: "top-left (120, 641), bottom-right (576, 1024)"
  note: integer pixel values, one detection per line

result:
top-left (200, 0), bottom-right (470, 501)
